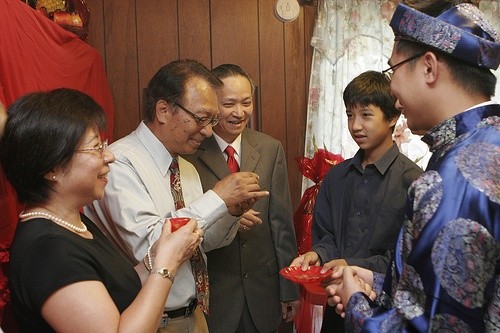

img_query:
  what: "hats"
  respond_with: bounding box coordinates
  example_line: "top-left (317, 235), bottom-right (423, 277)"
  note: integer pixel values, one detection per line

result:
top-left (389, 3), bottom-right (500, 71)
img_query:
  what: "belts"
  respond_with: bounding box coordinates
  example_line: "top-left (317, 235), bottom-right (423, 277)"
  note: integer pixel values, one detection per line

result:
top-left (164, 298), bottom-right (198, 319)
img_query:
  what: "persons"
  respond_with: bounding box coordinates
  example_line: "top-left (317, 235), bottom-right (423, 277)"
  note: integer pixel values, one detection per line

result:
top-left (325, 0), bottom-right (500, 333)
top-left (0, 89), bottom-right (204, 333)
top-left (86, 59), bottom-right (269, 333)
top-left (290, 71), bottom-right (425, 333)
top-left (180, 65), bottom-right (300, 333)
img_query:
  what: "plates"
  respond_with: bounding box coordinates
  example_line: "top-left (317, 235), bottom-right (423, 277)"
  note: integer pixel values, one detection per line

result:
top-left (279, 265), bottom-right (333, 283)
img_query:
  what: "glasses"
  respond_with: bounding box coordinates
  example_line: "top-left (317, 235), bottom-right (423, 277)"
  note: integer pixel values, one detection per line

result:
top-left (382, 50), bottom-right (440, 82)
top-left (73, 139), bottom-right (108, 157)
top-left (175, 102), bottom-right (221, 127)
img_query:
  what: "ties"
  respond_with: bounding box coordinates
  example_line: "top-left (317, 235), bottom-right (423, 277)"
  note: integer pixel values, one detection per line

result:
top-left (169, 158), bottom-right (210, 316)
top-left (224, 145), bottom-right (240, 175)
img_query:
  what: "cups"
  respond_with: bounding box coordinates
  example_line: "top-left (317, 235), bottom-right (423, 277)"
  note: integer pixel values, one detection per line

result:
top-left (170, 218), bottom-right (190, 233)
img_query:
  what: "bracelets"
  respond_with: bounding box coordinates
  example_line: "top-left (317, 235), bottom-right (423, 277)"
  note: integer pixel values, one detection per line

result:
top-left (148, 245), bottom-right (152, 269)
top-left (143, 259), bottom-right (150, 271)
top-left (150, 268), bottom-right (174, 284)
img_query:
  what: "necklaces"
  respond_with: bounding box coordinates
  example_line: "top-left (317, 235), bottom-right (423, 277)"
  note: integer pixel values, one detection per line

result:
top-left (18, 210), bottom-right (88, 232)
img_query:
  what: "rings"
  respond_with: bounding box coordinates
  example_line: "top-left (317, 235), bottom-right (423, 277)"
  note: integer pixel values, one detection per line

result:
top-left (195, 231), bottom-right (200, 238)
top-left (243, 226), bottom-right (247, 230)
